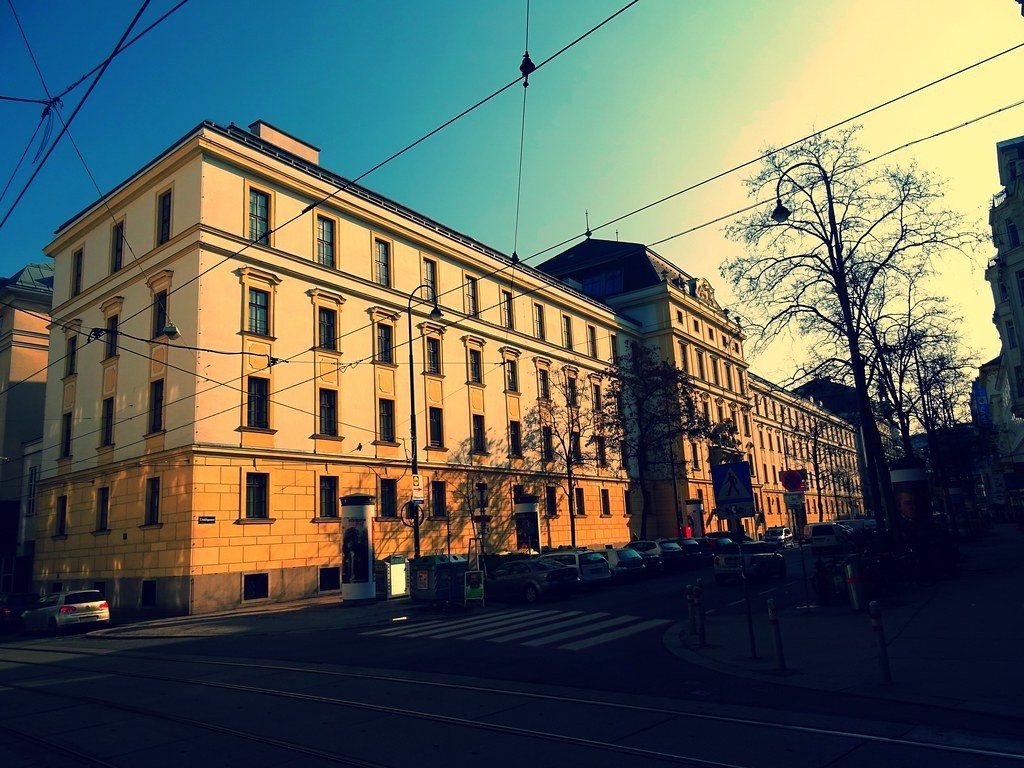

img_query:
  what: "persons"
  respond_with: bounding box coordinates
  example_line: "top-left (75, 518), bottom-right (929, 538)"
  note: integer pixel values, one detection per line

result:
top-left (466, 573), bottom-right (483, 598)
top-left (895, 486), bottom-right (917, 522)
top-left (343, 516), bottom-right (369, 582)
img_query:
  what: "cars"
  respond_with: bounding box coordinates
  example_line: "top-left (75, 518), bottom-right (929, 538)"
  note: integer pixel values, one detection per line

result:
top-left (488, 515), bottom-right (755, 602)
top-left (0, 593), bottom-right (34, 636)
top-left (15, 588), bottom-right (110, 637)
top-left (712, 536), bottom-right (787, 587)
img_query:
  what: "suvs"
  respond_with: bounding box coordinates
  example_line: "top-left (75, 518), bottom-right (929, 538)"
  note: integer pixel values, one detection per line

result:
top-left (763, 525), bottom-right (794, 549)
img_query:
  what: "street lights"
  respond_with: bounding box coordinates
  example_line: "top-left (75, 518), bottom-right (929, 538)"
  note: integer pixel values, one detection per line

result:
top-left (406, 283), bottom-right (445, 609)
top-left (771, 160), bottom-right (897, 575)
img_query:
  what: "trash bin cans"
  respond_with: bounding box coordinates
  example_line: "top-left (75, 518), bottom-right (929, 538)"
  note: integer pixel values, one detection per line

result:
top-left (844, 559), bottom-right (871, 612)
top-left (408, 553), bottom-right (469, 609)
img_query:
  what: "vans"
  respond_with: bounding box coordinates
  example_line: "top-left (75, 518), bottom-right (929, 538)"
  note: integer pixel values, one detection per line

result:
top-left (811, 523), bottom-right (850, 550)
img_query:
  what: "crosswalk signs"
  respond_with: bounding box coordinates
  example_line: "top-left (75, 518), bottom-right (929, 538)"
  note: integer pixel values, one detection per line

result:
top-left (710, 462), bottom-right (756, 520)
